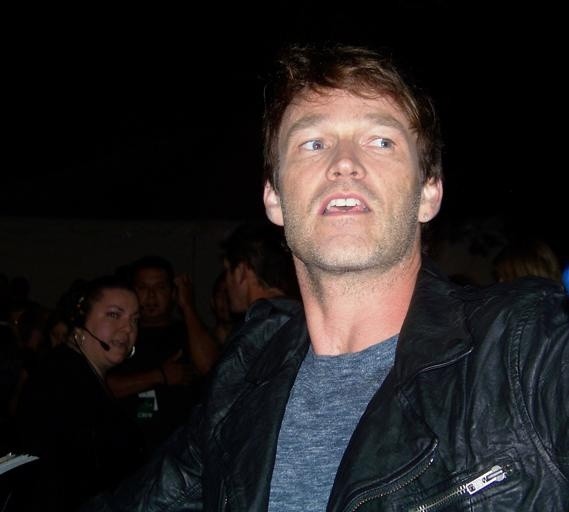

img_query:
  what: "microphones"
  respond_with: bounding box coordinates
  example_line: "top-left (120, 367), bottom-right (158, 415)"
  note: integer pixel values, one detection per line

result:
top-left (80, 322), bottom-right (112, 353)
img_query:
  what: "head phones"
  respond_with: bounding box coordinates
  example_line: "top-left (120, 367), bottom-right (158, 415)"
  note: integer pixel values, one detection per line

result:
top-left (68, 275), bottom-right (110, 330)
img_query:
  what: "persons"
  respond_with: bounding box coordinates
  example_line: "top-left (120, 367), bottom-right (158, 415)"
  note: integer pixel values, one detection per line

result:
top-left (80, 39), bottom-right (569, 510)
top-left (419, 208), bottom-right (569, 298)
top-left (1, 223), bottom-right (296, 511)
